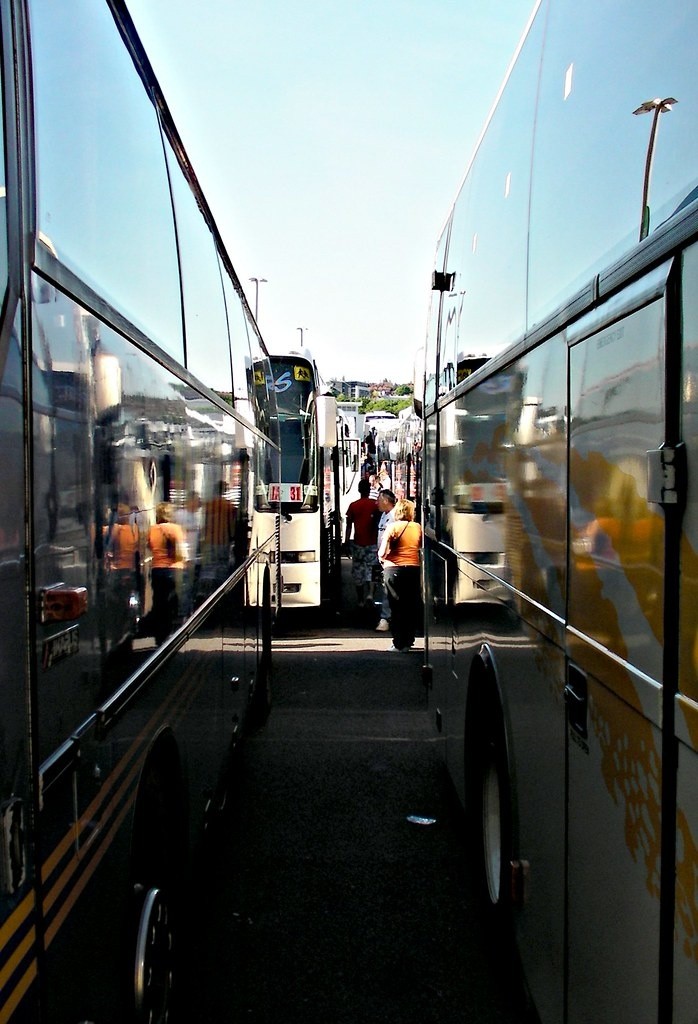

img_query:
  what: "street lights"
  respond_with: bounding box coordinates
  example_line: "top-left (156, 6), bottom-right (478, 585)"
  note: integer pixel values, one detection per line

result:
top-left (248, 276), bottom-right (267, 321)
top-left (631, 95), bottom-right (677, 240)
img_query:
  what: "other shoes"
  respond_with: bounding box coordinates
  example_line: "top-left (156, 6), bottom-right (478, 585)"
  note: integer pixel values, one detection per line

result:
top-left (366, 595), bottom-right (376, 610)
top-left (357, 600), bottom-right (365, 607)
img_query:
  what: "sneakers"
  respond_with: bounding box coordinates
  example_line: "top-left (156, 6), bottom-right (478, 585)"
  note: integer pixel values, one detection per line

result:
top-left (376, 618), bottom-right (390, 632)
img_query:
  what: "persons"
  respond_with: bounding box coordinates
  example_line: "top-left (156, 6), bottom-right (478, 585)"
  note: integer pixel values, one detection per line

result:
top-left (99, 478), bottom-right (239, 649)
top-left (345, 470), bottom-right (423, 652)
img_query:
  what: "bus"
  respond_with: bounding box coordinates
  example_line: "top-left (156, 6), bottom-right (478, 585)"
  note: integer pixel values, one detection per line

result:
top-left (0, 0), bottom-right (697, 1022)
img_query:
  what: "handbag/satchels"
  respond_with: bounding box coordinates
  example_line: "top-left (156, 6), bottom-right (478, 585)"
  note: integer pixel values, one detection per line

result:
top-left (382, 523), bottom-right (410, 558)
